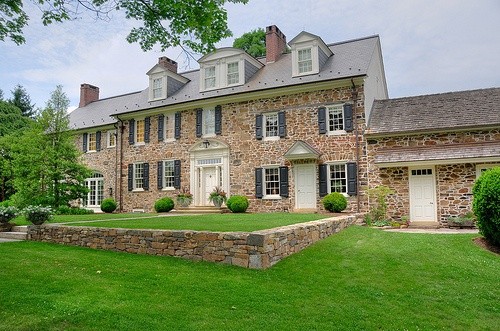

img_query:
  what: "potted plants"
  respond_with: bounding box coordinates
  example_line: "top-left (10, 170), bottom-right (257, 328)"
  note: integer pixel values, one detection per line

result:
top-left (207, 186), bottom-right (228, 207)
top-left (448, 211), bottom-right (476, 229)
top-left (176, 186), bottom-right (193, 207)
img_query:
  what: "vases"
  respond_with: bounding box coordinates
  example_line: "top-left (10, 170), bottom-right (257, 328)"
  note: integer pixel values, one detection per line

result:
top-left (1, 217), bottom-right (9, 223)
top-left (31, 218), bottom-right (45, 225)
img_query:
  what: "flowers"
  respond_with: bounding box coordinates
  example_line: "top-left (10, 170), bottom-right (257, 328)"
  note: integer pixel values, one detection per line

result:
top-left (22, 203), bottom-right (56, 219)
top-left (0, 205), bottom-right (21, 217)
